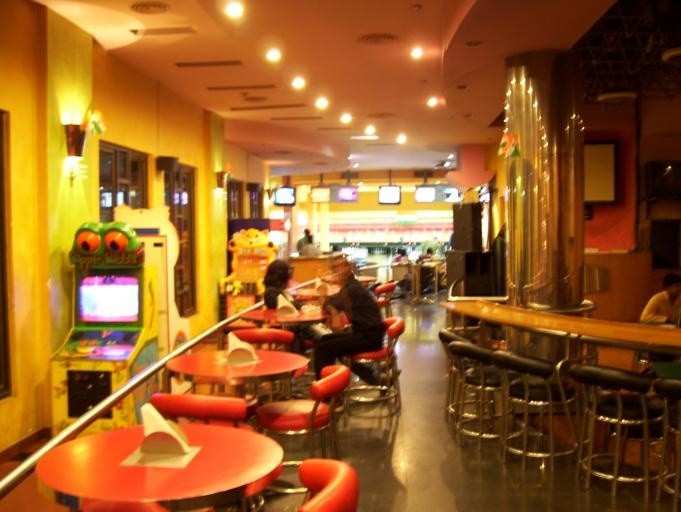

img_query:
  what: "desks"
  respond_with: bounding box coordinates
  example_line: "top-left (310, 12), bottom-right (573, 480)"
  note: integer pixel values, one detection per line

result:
top-left (294, 289), bottom-right (339, 304)
top-left (438, 299), bottom-right (681, 373)
top-left (240, 308), bottom-right (330, 351)
top-left (163, 351), bottom-right (311, 403)
top-left (35, 425), bottom-right (285, 512)
top-left (321, 276), bottom-right (377, 291)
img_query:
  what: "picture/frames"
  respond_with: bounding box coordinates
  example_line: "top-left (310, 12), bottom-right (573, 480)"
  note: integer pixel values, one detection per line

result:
top-left (584, 140), bottom-right (619, 205)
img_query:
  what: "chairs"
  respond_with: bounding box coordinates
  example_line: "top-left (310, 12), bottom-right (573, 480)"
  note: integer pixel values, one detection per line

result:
top-left (254, 365), bottom-right (352, 493)
top-left (374, 283), bottom-right (396, 318)
top-left (342, 317), bottom-right (406, 410)
top-left (230, 329), bottom-right (295, 422)
top-left (438, 330), bottom-right (681, 512)
top-left (149, 393), bottom-right (248, 428)
top-left (297, 459), bottom-right (360, 512)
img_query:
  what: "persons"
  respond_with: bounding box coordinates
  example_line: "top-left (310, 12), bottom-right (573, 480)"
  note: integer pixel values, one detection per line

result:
top-left (314, 257), bottom-right (387, 402)
top-left (302, 235), bottom-right (321, 257)
top-left (639, 272), bottom-right (681, 363)
top-left (424, 248), bottom-right (434, 258)
top-left (395, 247), bottom-right (406, 262)
top-left (297, 229), bottom-right (310, 256)
top-left (263, 260), bottom-right (401, 396)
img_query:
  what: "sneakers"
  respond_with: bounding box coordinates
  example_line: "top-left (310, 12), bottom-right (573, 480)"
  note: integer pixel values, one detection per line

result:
top-left (380, 369), bottom-right (402, 394)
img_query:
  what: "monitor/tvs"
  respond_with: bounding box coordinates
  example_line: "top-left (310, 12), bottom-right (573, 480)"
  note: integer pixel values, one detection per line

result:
top-left (275, 187), bottom-right (294, 203)
top-left (337, 186), bottom-right (358, 201)
top-left (78, 275), bottom-right (139, 323)
top-left (378, 187), bottom-right (400, 204)
top-left (311, 187), bottom-right (330, 202)
top-left (442, 187), bottom-right (462, 203)
top-left (415, 185), bottom-right (435, 202)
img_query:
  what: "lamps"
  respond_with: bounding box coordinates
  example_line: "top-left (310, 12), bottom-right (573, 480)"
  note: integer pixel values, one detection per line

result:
top-left (217, 170), bottom-right (233, 196)
top-left (65, 111), bottom-right (107, 186)
